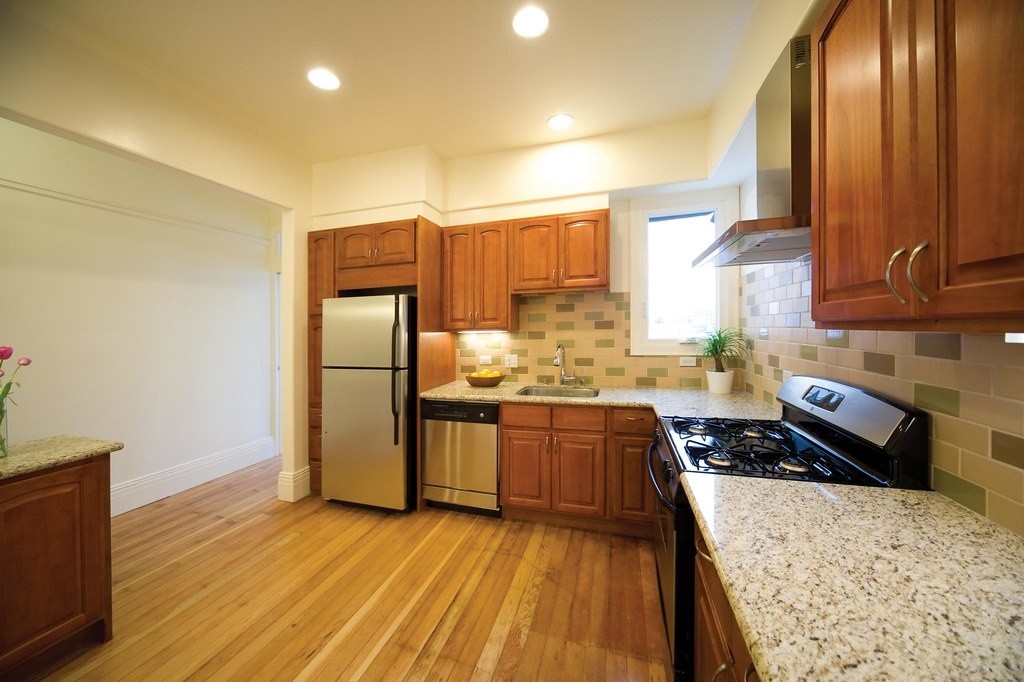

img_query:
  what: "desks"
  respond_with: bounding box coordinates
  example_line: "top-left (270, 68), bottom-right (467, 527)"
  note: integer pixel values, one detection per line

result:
top-left (0, 436), bottom-right (126, 682)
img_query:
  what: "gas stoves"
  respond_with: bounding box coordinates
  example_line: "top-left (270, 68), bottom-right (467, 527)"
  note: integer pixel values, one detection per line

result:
top-left (651, 374), bottom-right (929, 508)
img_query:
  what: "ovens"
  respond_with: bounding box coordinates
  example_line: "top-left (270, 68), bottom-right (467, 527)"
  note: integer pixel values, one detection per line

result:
top-left (647, 442), bottom-right (695, 682)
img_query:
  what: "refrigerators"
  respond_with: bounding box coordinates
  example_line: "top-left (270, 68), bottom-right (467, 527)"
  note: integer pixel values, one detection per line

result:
top-left (322, 294), bottom-right (417, 513)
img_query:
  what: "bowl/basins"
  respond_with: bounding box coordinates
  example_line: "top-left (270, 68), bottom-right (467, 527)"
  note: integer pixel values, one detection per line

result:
top-left (465, 373), bottom-right (506, 387)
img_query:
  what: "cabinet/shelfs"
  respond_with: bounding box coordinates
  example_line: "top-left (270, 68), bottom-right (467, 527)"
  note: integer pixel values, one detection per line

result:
top-left (509, 207), bottom-right (610, 295)
top-left (499, 400), bottom-right (606, 519)
top-left (307, 216), bottom-right (456, 511)
top-left (810, 0), bottom-right (1024, 335)
top-left (693, 522), bottom-right (763, 682)
top-left (443, 220), bottom-right (520, 332)
top-left (609, 407), bottom-right (656, 521)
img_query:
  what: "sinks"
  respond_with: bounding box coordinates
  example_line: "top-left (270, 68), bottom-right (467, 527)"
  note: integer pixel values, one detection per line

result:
top-left (516, 385), bottom-right (601, 398)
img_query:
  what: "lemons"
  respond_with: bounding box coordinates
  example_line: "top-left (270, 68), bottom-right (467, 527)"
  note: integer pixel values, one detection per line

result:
top-left (471, 369), bottom-right (502, 378)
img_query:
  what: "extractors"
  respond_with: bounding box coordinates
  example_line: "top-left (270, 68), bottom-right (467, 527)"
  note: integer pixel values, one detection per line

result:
top-left (692, 34), bottom-right (811, 268)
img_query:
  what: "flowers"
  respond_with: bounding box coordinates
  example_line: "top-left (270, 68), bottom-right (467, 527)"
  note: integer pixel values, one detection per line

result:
top-left (0, 345), bottom-right (34, 455)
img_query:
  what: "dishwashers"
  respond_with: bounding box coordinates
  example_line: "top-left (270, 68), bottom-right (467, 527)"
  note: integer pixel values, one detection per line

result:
top-left (422, 397), bottom-right (502, 518)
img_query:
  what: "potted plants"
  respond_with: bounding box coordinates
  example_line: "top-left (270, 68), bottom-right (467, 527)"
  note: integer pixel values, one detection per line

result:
top-left (694, 326), bottom-right (752, 395)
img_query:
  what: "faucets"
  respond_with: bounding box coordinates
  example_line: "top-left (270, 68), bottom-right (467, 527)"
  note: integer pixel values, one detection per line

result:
top-left (553, 343), bottom-right (577, 388)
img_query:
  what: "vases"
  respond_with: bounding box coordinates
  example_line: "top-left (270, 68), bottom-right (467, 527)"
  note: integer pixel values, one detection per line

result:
top-left (0, 395), bottom-right (8, 458)
